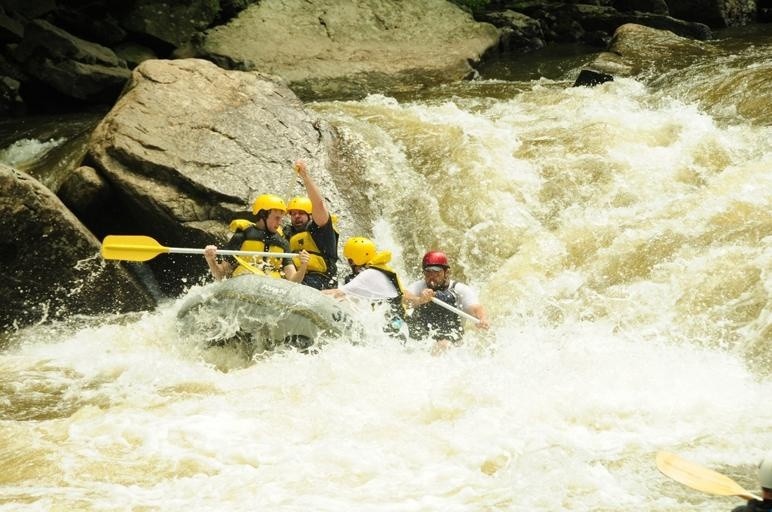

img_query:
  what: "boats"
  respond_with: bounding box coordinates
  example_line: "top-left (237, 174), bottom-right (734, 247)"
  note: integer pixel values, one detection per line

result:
top-left (171, 274), bottom-right (383, 363)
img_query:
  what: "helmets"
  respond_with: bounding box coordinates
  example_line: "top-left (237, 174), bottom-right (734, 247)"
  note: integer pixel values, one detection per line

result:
top-left (342, 236), bottom-right (379, 266)
top-left (251, 194), bottom-right (286, 216)
top-left (421, 250), bottom-right (452, 271)
top-left (287, 196), bottom-right (314, 215)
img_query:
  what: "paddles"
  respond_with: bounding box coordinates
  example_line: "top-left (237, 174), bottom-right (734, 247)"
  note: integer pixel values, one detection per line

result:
top-left (655, 450), bottom-right (762, 502)
top-left (101, 236), bottom-right (299, 262)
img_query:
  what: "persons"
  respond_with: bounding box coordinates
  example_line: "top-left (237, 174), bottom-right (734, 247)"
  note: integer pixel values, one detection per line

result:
top-left (283, 158), bottom-right (340, 296)
top-left (320, 237), bottom-right (409, 348)
top-left (204, 195), bottom-right (310, 283)
top-left (403, 252), bottom-right (489, 356)
top-left (728, 458), bottom-right (772, 512)
